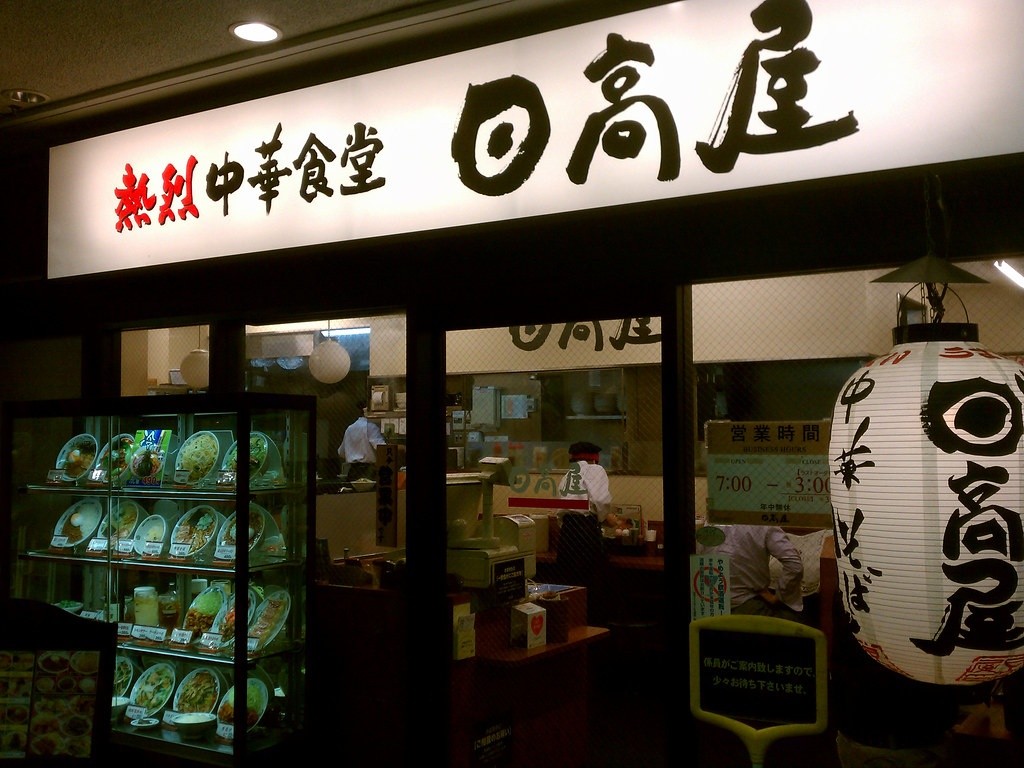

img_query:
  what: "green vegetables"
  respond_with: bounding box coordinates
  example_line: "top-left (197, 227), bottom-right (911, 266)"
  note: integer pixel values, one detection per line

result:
top-left (134, 450), bottom-right (154, 477)
top-left (114, 661), bottom-right (131, 697)
top-left (133, 666), bottom-right (174, 709)
top-left (73, 440), bottom-right (96, 457)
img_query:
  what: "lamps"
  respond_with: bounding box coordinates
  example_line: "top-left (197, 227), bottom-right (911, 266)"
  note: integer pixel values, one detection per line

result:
top-left (308, 318), bottom-right (351, 384)
top-left (181, 325), bottom-right (210, 390)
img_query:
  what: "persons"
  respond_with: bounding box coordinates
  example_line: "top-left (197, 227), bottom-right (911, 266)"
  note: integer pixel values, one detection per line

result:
top-left (556, 442), bottom-right (613, 591)
top-left (699, 523), bottom-right (805, 617)
top-left (338, 401), bottom-right (386, 482)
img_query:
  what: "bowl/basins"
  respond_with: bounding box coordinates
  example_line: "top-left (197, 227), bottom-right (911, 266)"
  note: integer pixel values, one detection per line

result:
top-left (53, 601), bottom-right (83, 613)
top-left (112, 696), bottom-right (129, 719)
top-left (135, 514), bottom-right (166, 555)
top-left (395, 392), bottom-right (406, 409)
top-left (171, 712), bottom-right (217, 739)
top-left (571, 392), bottom-right (590, 416)
top-left (96, 433), bottom-right (135, 483)
top-left (55, 433), bottom-right (98, 483)
top-left (448, 393), bottom-right (461, 405)
top-left (351, 481), bottom-right (376, 492)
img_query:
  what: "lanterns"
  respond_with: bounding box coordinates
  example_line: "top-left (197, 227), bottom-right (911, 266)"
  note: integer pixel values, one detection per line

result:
top-left (179, 348), bottom-right (209, 389)
top-left (827, 320), bottom-right (1023, 704)
top-left (309, 340), bottom-right (350, 384)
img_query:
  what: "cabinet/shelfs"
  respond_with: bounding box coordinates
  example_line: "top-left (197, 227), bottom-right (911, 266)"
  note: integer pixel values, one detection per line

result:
top-left (1, 391), bottom-right (318, 768)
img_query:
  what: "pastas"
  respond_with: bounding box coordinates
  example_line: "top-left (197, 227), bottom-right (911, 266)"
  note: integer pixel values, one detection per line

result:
top-left (178, 434), bottom-right (217, 483)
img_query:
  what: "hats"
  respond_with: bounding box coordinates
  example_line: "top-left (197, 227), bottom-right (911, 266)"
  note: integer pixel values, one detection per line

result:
top-left (570, 442), bottom-right (602, 460)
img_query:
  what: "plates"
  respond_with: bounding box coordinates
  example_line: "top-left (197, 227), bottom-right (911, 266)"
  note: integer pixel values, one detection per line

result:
top-left (222, 432), bottom-right (282, 481)
top-left (97, 501), bottom-right (139, 550)
top-left (0, 650), bottom-right (100, 760)
top-left (53, 497), bottom-right (103, 548)
top-left (114, 655), bottom-right (275, 736)
top-left (170, 504), bottom-right (280, 560)
top-left (183, 586), bottom-right (291, 655)
top-left (130, 718), bottom-right (160, 729)
top-left (174, 431), bottom-right (220, 486)
top-left (591, 390), bottom-right (626, 414)
top-left (131, 446), bottom-right (163, 479)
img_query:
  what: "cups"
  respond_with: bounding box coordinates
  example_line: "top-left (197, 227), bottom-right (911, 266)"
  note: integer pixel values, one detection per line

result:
top-left (210, 579), bottom-right (231, 597)
top-left (536, 595), bottom-right (570, 645)
top-left (191, 578), bottom-right (207, 600)
top-left (123, 586), bottom-right (179, 637)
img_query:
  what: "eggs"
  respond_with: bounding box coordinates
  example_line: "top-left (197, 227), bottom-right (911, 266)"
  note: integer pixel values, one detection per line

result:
top-left (71, 513), bottom-right (83, 527)
